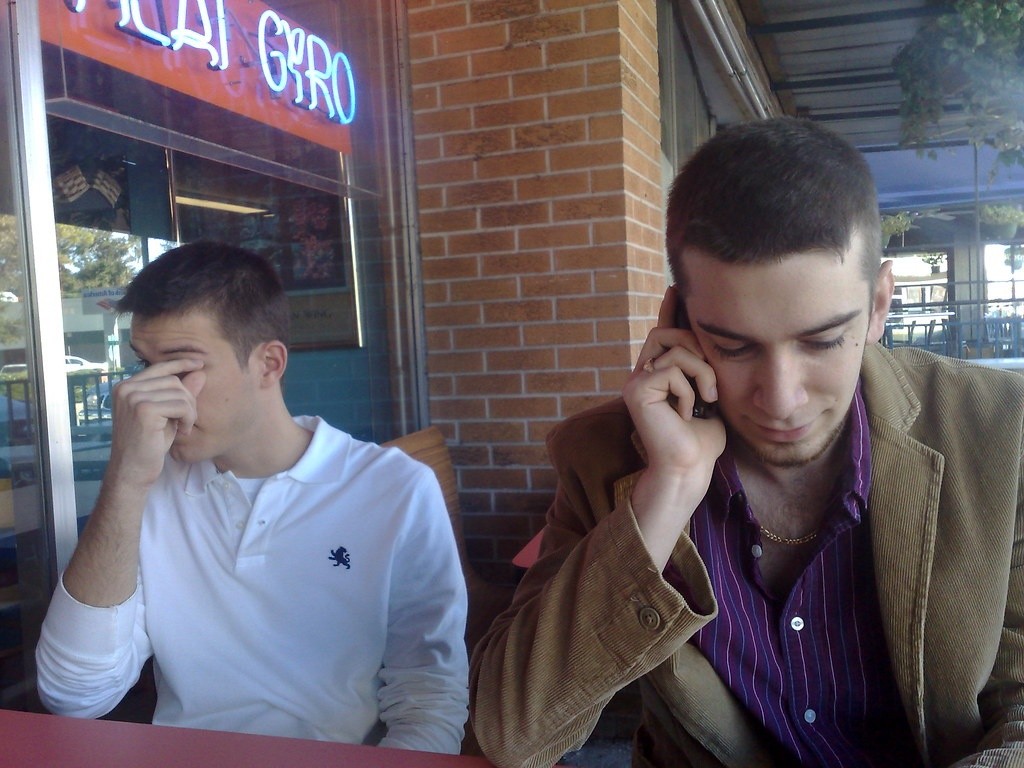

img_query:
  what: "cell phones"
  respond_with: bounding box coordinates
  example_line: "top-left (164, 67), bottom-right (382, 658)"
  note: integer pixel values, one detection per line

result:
top-left (672, 300), bottom-right (710, 419)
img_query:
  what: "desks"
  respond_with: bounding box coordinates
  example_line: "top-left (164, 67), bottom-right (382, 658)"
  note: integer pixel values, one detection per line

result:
top-left (0, 708), bottom-right (501, 768)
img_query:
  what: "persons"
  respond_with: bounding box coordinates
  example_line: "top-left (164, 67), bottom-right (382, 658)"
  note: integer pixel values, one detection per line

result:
top-left (36, 237), bottom-right (468, 755)
top-left (469, 114), bottom-right (1024, 768)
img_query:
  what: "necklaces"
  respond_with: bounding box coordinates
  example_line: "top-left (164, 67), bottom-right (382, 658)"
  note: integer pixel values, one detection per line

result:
top-left (761, 525), bottom-right (818, 545)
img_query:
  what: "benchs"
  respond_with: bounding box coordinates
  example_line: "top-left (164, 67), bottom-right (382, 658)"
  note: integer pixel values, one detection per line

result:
top-left (378, 428), bottom-right (521, 656)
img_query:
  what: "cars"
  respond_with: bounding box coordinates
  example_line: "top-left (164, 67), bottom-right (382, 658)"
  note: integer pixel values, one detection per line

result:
top-left (0, 355), bottom-right (135, 486)
